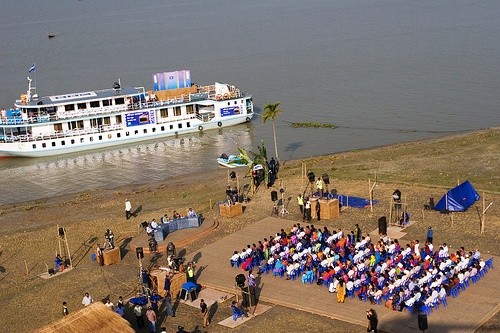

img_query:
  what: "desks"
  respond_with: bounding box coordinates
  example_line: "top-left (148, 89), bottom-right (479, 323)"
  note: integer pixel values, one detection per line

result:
top-left (153, 216), bottom-right (201, 241)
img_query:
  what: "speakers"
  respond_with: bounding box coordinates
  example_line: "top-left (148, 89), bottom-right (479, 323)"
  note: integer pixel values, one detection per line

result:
top-left (136, 247), bottom-right (143, 259)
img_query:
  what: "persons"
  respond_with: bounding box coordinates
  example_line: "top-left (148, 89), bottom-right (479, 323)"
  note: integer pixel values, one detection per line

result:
top-left (146, 218), bottom-right (158, 235)
top-left (367, 309), bottom-right (378, 333)
top-left (297, 193), bottom-right (320, 222)
top-left (188, 208), bottom-right (197, 217)
top-left (82, 292), bottom-right (93, 307)
top-left (252, 157), bottom-right (275, 186)
top-left (226, 171), bottom-right (238, 205)
top-left (0, 108), bottom-right (7, 124)
top-left (173, 211), bottom-right (181, 219)
top-left (125, 199), bottom-right (134, 220)
top-left (316, 177), bottom-right (323, 199)
top-left (55, 255), bottom-right (65, 272)
top-left (62, 302), bottom-right (68, 316)
top-left (96, 244), bottom-right (103, 266)
top-left (105, 255), bottom-right (209, 333)
top-left (163, 214), bottom-right (169, 223)
top-left (229, 223), bottom-right (486, 321)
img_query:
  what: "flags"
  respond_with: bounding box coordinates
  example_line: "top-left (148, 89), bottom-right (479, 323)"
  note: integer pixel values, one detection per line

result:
top-left (29, 64), bottom-right (35, 72)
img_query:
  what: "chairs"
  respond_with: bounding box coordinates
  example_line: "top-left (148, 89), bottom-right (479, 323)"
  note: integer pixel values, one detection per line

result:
top-left (231, 227), bottom-right (493, 315)
top-left (179, 281), bottom-right (200, 301)
top-left (232, 307), bottom-right (244, 321)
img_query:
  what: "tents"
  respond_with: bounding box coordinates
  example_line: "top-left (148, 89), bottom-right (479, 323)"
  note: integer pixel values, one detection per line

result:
top-left (435, 180), bottom-right (480, 212)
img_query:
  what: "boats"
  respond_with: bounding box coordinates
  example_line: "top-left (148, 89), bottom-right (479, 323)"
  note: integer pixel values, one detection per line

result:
top-left (0, 64), bottom-right (255, 158)
